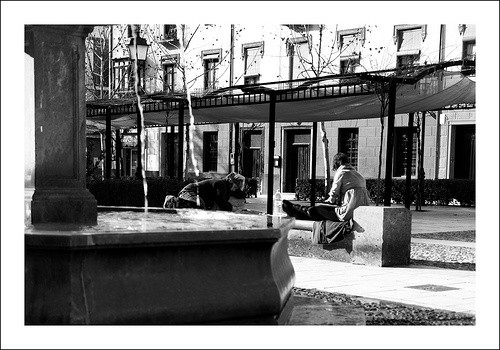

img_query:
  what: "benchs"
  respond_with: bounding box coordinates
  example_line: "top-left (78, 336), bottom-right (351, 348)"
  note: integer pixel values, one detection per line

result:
top-left (216, 206), bottom-right (413, 270)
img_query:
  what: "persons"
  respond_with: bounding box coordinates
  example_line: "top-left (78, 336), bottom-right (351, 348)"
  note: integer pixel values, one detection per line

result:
top-left (163, 176), bottom-right (241, 209)
top-left (282, 153), bottom-right (369, 223)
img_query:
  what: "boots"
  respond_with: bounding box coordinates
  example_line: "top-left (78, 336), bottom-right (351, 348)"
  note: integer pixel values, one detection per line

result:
top-left (282, 204), bottom-right (307, 217)
top-left (283, 200), bottom-right (313, 218)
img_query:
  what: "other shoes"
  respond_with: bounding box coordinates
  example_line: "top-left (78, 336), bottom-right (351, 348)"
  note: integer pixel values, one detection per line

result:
top-left (164, 195), bottom-right (178, 209)
